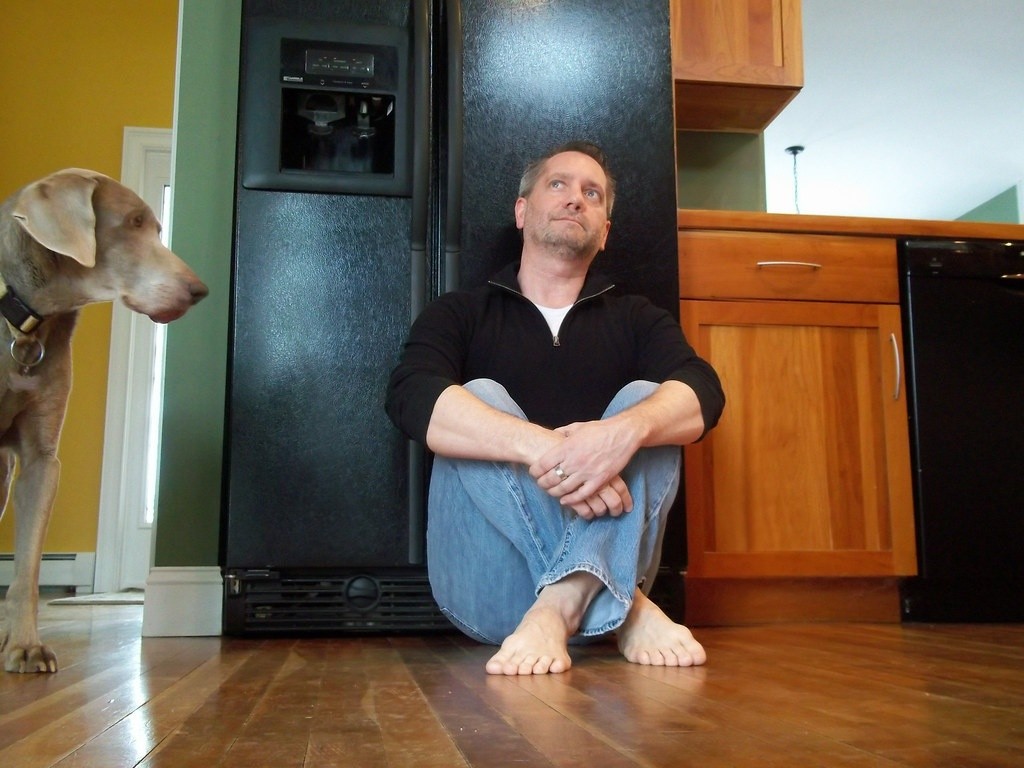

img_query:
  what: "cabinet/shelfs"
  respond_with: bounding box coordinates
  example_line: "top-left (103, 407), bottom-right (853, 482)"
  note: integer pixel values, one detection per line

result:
top-left (669, 1), bottom-right (805, 134)
top-left (675, 209), bottom-right (921, 629)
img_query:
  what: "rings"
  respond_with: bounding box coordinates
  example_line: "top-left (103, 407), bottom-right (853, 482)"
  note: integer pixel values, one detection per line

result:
top-left (555, 466), bottom-right (568, 481)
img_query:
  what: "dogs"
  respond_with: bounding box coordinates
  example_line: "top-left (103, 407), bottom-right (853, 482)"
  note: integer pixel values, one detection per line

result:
top-left (0, 168), bottom-right (208, 673)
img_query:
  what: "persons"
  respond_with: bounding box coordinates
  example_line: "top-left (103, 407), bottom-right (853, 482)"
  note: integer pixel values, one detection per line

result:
top-left (381, 145), bottom-right (728, 677)
